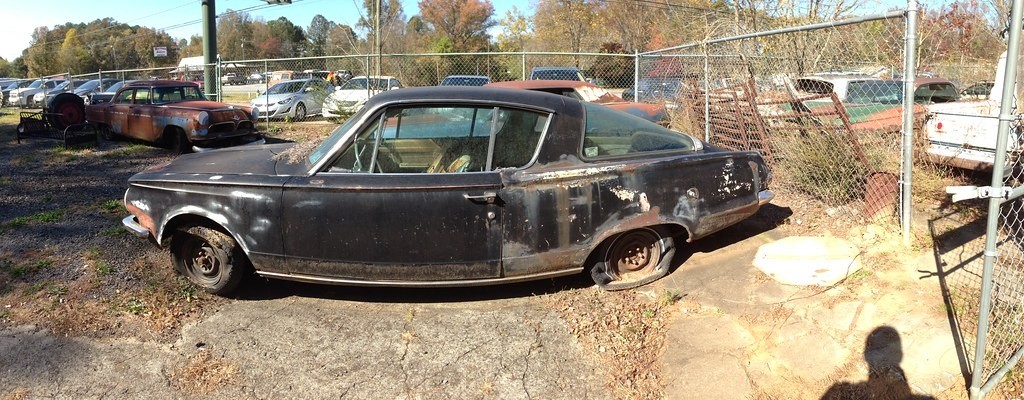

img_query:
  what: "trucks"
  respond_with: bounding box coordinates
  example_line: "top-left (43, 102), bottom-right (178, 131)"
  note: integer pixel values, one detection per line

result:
top-left (218, 73), bottom-right (246, 85)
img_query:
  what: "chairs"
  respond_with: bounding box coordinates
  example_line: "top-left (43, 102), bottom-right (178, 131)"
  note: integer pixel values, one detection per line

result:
top-left (426, 137), bottom-right (476, 173)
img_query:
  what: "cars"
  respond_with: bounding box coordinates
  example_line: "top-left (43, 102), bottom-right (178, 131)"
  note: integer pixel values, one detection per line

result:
top-left (84, 80), bottom-right (260, 153)
top-left (374, 80), bottom-right (671, 139)
top-left (9, 79), bottom-right (67, 108)
top-left (427, 75), bottom-right (491, 114)
top-left (2, 81), bottom-right (34, 107)
top-left (779, 51), bottom-right (1021, 174)
top-left (122, 86), bottom-right (776, 296)
top-left (67, 78), bottom-right (123, 96)
top-left (622, 79), bottom-right (681, 105)
top-left (33, 79), bottom-right (89, 108)
top-left (322, 76), bottom-right (403, 120)
top-left (246, 73), bottom-right (270, 84)
top-left (527, 66), bottom-right (587, 81)
top-left (87, 80), bottom-right (136, 104)
top-left (333, 70), bottom-right (350, 78)
top-left (249, 78), bottom-right (335, 121)
